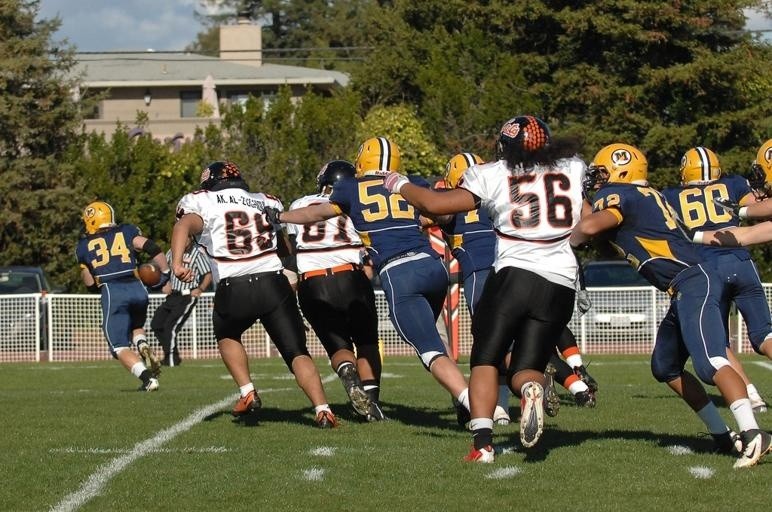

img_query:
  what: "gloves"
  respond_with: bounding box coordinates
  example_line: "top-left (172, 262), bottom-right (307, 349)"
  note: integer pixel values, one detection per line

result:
top-left (714, 196), bottom-right (747, 220)
top-left (675, 215), bottom-right (703, 244)
top-left (152, 269), bottom-right (171, 290)
top-left (261, 206), bottom-right (281, 227)
top-left (382, 172), bottom-right (409, 193)
top-left (577, 289), bottom-right (592, 317)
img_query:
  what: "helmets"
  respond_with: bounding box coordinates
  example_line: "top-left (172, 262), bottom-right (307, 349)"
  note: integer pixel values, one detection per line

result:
top-left (582, 143), bottom-right (648, 199)
top-left (80, 201), bottom-right (117, 235)
top-left (315, 160), bottom-right (355, 195)
top-left (201, 162), bottom-right (241, 189)
top-left (746, 138), bottom-right (772, 201)
top-left (680, 147), bottom-right (721, 187)
top-left (355, 137), bottom-right (400, 178)
top-left (497, 116), bottom-right (553, 162)
top-left (444, 153), bottom-right (485, 189)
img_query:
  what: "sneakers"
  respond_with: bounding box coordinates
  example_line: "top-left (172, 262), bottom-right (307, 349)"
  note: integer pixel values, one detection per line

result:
top-left (456, 364), bottom-right (598, 464)
top-left (340, 363), bottom-right (384, 422)
top-left (230, 390), bottom-right (260, 416)
top-left (710, 396), bottom-right (772, 470)
top-left (136, 340), bottom-right (161, 391)
top-left (313, 410), bottom-right (336, 427)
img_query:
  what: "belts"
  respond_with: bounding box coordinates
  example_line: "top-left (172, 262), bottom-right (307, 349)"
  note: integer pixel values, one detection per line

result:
top-left (299, 264), bottom-right (359, 282)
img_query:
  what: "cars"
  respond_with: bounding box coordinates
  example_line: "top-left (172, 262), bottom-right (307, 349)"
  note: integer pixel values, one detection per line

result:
top-left (574, 257), bottom-right (669, 341)
top-left (0, 265), bottom-right (67, 350)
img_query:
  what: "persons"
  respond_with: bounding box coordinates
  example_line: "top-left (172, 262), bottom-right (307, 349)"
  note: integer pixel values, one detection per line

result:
top-left (170, 161), bottom-right (340, 428)
top-left (569, 144), bottom-right (770, 468)
top-left (76, 201), bottom-right (170, 393)
top-left (261, 137), bottom-right (471, 432)
top-left (661, 146), bottom-right (771, 416)
top-left (383, 116), bottom-right (595, 464)
top-left (286, 162), bottom-right (383, 421)
top-left (150, 233), bottom-right (212, 368)
top-left (677, 139), bottom-right (771, 249)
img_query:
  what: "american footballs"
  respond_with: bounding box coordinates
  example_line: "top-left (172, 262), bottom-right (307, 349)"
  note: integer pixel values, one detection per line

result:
top-left (138, 263), bottom-right (160, 285)
